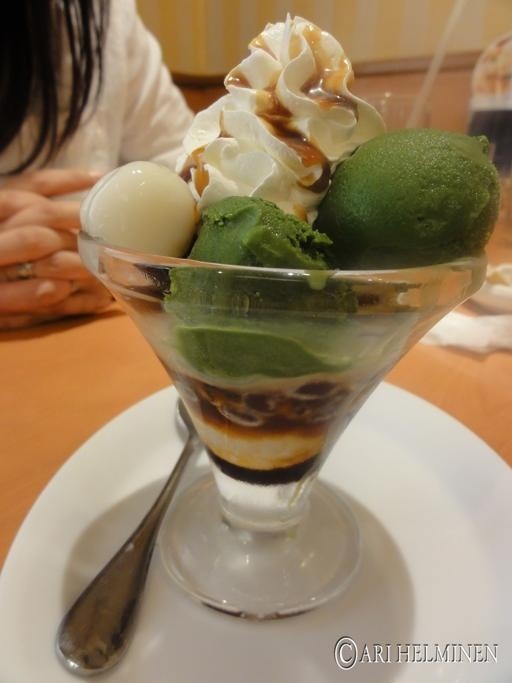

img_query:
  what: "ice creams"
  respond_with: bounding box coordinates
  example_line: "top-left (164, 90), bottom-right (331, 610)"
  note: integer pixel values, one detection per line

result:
top-left (77, 13), bottom-right (501, 512)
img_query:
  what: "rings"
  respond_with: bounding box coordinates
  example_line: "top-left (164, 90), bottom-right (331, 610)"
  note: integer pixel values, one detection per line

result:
top-left (14, 261), bottom-right (36, 277)
top-left (66, 277), bottom-right (80, 292)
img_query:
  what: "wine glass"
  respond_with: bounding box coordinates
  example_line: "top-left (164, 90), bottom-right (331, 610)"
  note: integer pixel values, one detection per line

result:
top-left (68, 228), bottom-right (492, 623)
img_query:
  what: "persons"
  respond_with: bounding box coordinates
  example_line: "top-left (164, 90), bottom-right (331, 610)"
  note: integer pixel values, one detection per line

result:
top-left (0, 0), bottom-right (200, 325)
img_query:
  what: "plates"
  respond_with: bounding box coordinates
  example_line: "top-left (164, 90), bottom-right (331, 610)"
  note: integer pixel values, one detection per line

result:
top-left (0, 377), bottom-right (511, 682)
